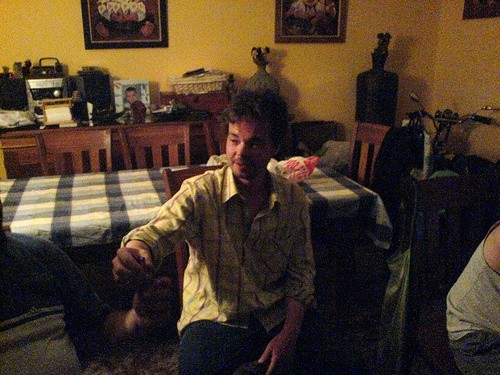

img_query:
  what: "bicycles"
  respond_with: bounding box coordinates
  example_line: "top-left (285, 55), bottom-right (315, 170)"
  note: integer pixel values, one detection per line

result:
top-left (400, 89), bottom-right (499, 173)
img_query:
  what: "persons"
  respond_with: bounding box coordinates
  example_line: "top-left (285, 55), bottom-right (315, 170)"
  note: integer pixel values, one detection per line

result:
top-left (0, 196), bottom-right (174, 375)
top-left (446, 219), bottom-right (500, 375)
top-left (112, 88), bottom-right (316, 375)
top-left (124, 87), bottom-right (137, 110)
top-left (131, 101), bottom-right (146, 123)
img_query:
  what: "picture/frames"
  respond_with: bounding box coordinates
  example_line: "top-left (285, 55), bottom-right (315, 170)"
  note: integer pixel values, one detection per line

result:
top-left (81, 0), bottom-right (170, 50)
top-left (113, 79), bottom-right (152, 123)
top-left (273, 0), bottom-right (350, 44)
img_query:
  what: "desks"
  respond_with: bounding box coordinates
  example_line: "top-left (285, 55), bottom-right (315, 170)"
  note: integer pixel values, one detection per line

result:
top-left (0, 159), bottom-right (395, 337)
top-left (0, 118), bottom-right (222, 180)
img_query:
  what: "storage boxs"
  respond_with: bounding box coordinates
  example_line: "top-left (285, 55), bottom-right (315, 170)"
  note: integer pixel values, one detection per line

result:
top-left (171, 69), bottom-right (235, 96)
top-left (68, 73), bottom-right (113, 122)
top-left (41, 97), bottom-right (76, 125)
top-left (0, 72), bottom-right (29, 111)
top-left (159, 91), bottom-right (230, 115)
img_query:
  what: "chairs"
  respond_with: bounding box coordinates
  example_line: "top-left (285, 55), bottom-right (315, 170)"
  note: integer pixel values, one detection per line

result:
top-left (202, 118), bottom-right (230, 156)
top-left (399, 158), bottom-right (500, 375)
top-left (291, 120), bottom-right (345, 158)
top-left (160, 163), bottom-right (226, 308)
top-left (117, 121), bottom-right (190, 169)
top-left (331, 121), bottom-right (391, 326)
top-left (33, 125), bottom-right (112, 177)
